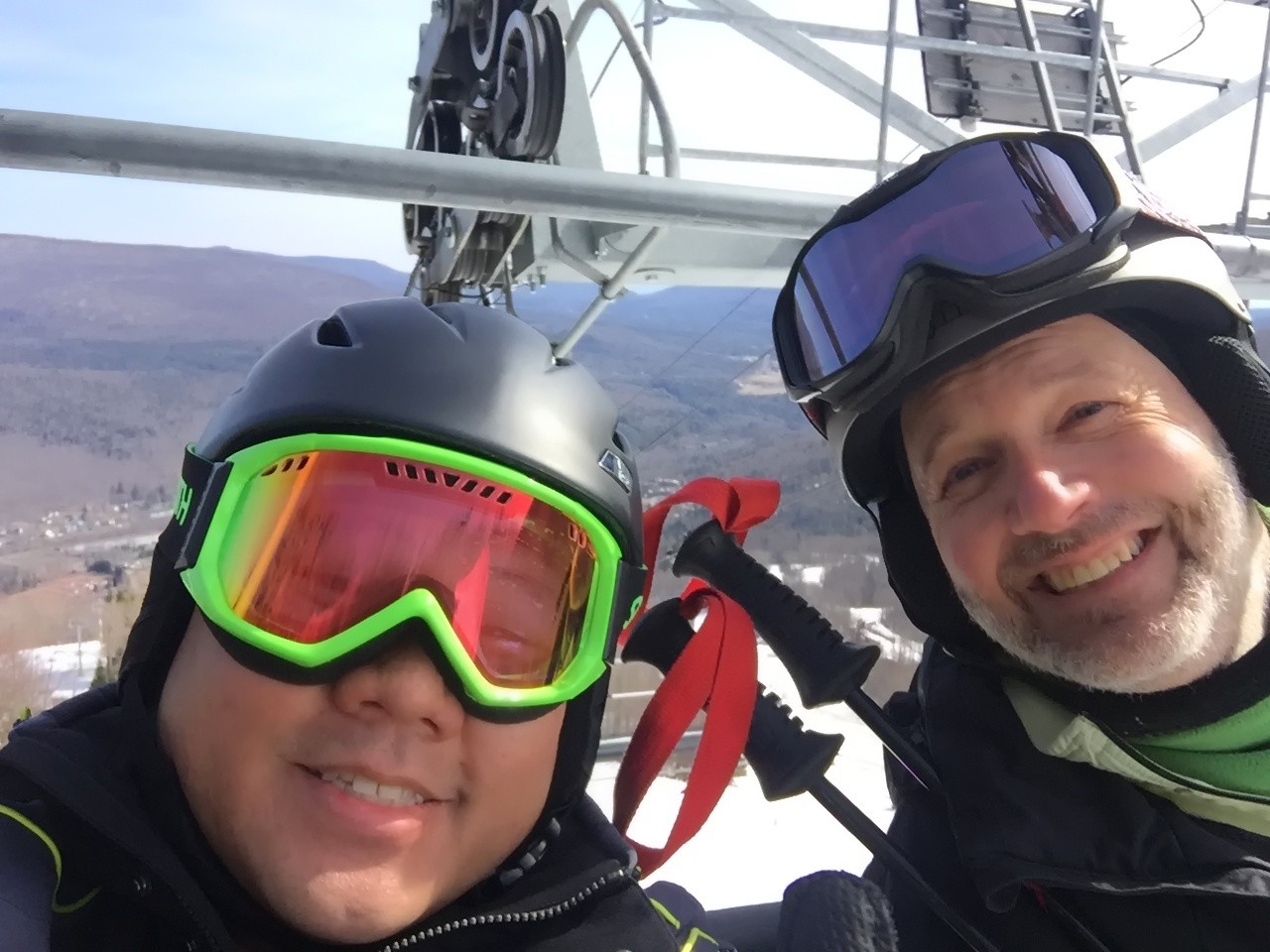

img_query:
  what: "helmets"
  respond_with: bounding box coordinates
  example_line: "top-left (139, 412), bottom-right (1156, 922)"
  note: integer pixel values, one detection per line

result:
top-left (159, 298), bottom-right (657, 802)
top-left (819, 166), bottom-right (1269, 659)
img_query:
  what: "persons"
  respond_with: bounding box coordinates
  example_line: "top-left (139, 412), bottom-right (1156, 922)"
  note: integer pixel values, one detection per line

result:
top-left (771, 130), bottom-right (1270, 952)
top-left (0, 296), bottom-right (737, 952)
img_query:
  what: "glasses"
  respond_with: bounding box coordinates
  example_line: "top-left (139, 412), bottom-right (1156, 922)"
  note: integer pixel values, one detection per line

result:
top-left (767, 125), bottom-right (1132, 434)
top-left (176, 433), bottom-right (628, 718)
